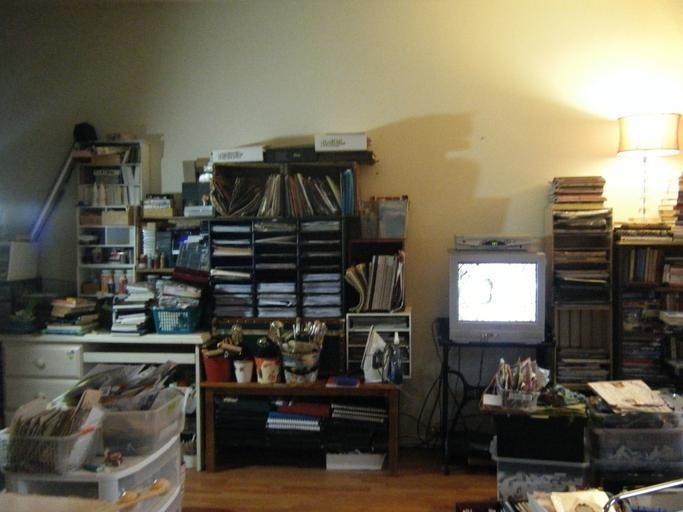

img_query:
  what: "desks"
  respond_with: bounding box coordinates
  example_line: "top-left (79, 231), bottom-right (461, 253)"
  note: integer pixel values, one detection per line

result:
top-left (436, 339), bottom-right (553, 476)
top-left (199, 380), bottom-right (400, 477)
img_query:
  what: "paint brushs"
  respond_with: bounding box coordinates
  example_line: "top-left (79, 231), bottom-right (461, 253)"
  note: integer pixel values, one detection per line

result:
top-left (293, 316), bottom-right (326, 346)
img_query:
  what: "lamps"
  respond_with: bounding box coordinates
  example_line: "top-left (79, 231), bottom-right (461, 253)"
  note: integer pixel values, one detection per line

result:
top-left (615, 112), bottom-right (680, 230)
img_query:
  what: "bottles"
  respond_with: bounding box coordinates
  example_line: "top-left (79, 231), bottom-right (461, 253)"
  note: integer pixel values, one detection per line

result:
top-left (98, 184), bottom-right (106, 205)
top-left (132, 184), bottom-right (139, 205)
top-left (138, 253), bottom-right (166, 269)
top-left (89, 182), bottom-right (97, 207)
top-left (390, 332), bottom-right (403, 385)
top-left (119, 185), bottom-right (129, 205)
top-left (100, 270), bottom-right (133, 293)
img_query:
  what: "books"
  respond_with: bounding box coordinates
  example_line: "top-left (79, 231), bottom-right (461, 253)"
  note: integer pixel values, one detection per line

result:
top-left (549, 174), bottom-right (683, 380)
top-left (111, 281), bottom-right (153, 336)
top-left (46, 297), bottom-right (100, 334)
top-left (207, 171), bottom-right (405, 316)
top-left (77, 146), bottom-right (138, 208)
top-left (218, 395), bottom-right (387, 430)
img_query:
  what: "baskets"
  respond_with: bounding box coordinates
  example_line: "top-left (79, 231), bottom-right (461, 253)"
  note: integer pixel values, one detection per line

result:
top-left (0, 424), bottom-right (99, 476)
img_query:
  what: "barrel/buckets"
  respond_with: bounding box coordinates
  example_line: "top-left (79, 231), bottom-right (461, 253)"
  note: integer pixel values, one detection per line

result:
top-left (253, 354), bottom-right (283, 385)
top-left (232, 359), bottom-right (254, 383)
top-left (279, 346), bottom-right (324, 386)
top-left (201, 349), bottom-right (236, 382)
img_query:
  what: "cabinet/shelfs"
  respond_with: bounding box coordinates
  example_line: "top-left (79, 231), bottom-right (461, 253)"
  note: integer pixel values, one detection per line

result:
top-left (542, 203), bottom-right (614, 392)
top-left (137, 160), bottom-right (345, 382)
top-left (1, 328), bottom-right (210, 472)
top-left (613, 241), bottom-right (681, 392)
top-left (347, 314), bottom-right (412, 381)
top-left (345, 239), bottom-right (407, 314)
top-left (71, 139), bottom-right (149, 295)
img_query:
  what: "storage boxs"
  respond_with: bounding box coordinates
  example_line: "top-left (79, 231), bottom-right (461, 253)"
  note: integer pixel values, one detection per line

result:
top-left (479, 387), bottom-right (682, 499)
top-left (0, 389), bottom-right (191, 512)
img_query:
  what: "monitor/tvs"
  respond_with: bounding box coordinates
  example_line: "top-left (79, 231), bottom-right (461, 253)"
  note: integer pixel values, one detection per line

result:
top-left (448, 249), bottom-right (547, 345)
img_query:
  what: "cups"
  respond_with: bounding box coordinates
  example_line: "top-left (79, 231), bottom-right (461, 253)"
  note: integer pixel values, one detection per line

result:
top-left (281, 352), bottom-right (319, 383)
top-left (234, 361), bottom-right (254, 382)
top-left (255, 356), bottom-right (280, 383)
top-left (202, 356), bottom-right (231, 380)
